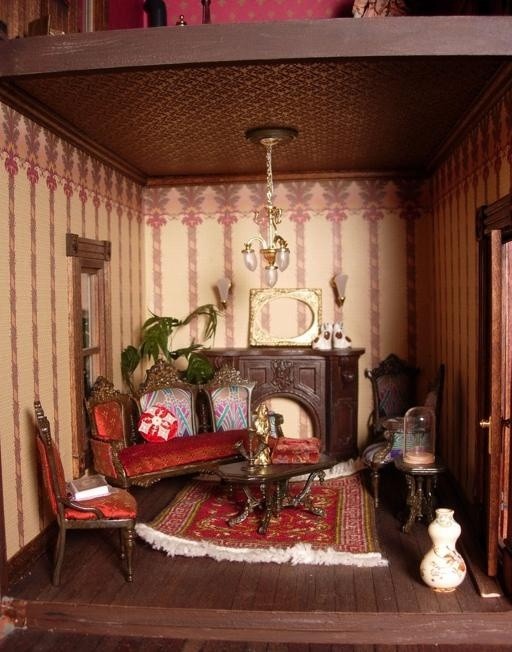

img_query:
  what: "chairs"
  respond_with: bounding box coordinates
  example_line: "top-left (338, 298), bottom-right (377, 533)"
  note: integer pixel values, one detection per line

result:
top-left (33, 401), bottom-right (137, 585)
top-left (362, 364), bottom-right (445, 508)
top-left (365, 353), bottom-right (420, 445)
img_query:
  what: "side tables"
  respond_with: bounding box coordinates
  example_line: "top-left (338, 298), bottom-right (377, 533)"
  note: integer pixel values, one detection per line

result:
top-left (395, 458), bottom-right (446, 533)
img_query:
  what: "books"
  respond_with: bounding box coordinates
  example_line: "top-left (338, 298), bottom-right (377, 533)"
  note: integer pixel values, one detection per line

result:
top-left (70, 474), bottom-right (109, 501)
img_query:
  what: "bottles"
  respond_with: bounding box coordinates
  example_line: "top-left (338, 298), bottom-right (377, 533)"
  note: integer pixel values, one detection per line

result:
top-left (419, 508), bottom-right (467, 594)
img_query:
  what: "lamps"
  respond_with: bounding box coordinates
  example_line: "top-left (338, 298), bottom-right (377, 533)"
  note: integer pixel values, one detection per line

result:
top-left (333, 272), bottom-right (348, 307)
top-left (242, 128), bottom-right (298, 288)
top-left (217, 277), bottom-right (231, 309)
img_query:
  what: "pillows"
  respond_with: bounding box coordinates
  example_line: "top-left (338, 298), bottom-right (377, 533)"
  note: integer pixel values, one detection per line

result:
top-left (138, 405), bottom-right (179, 442)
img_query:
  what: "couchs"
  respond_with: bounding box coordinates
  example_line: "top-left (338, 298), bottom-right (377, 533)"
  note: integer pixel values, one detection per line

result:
top-left (86, 359), bottom-right (258, 493)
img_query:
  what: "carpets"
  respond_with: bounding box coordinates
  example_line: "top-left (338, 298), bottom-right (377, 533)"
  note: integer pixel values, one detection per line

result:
top-left (134, 457), bottom-right (388, 567)
